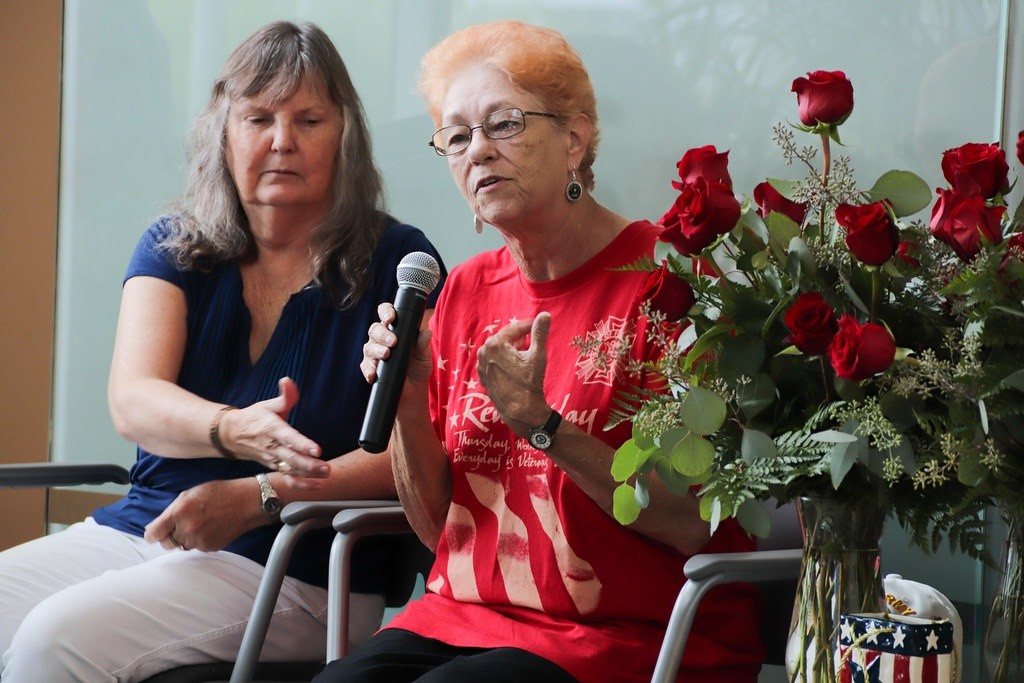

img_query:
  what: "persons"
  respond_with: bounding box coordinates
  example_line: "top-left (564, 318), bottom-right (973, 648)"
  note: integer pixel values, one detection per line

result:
top-left (313, 20), bottom-right (764, 683)
top-left (0, 22), bottom-right (448, 683)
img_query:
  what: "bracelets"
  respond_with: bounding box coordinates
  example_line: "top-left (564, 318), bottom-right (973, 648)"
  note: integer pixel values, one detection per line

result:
top-left (210, 405), bottom-right (236, 457)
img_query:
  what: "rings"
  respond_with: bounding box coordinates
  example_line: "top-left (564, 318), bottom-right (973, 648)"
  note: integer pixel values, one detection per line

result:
top-left (179, 545), bottom-right (190, 551)
top-left (169, 533), bottom-right (180, 547)
top-left (278, 462), bottom-right (292, 472)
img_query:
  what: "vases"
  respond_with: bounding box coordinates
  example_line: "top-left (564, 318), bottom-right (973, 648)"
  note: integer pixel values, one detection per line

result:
top-left (784, 498), bottom-right (887, 683)
top-left (982, 497), bottom-right (1024, 683)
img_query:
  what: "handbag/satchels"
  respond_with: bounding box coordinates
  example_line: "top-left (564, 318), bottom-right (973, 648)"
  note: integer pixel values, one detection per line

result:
top-left (836, 572), bottom-right (961, 683)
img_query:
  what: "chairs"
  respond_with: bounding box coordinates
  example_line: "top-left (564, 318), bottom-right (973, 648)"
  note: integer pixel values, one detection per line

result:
top-left (0, 463), bottom-right (806, 683)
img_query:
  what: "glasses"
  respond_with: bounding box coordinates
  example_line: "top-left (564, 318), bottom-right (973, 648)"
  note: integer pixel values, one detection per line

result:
top-left (428, 106), bottom-right (561, 157)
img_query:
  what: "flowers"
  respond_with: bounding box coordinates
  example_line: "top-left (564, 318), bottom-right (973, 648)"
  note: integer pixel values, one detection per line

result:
top-left (572, 71), bottom-right (1023, 683)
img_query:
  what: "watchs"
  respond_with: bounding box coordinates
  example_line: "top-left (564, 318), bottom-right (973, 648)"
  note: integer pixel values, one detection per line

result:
top-left (528, 411), bottom-right (563, 450)
top-left (255, 473), bottom-right (283, 522)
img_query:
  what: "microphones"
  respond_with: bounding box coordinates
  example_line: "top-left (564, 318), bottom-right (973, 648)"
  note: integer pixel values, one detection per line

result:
top-left (358, 252), bottom-right (440, 454)
